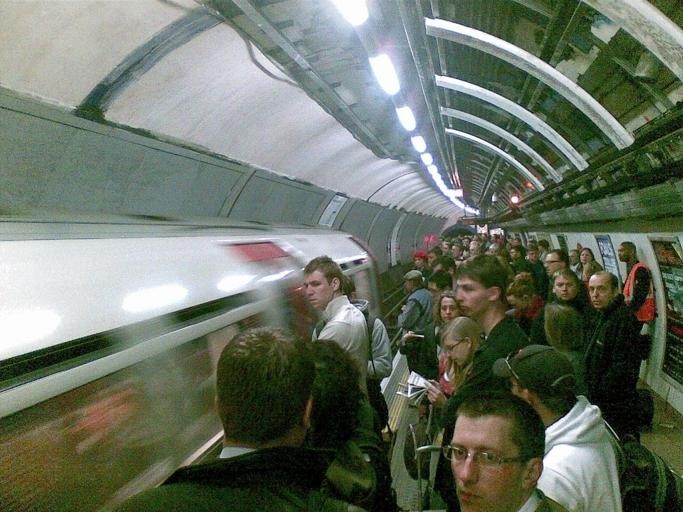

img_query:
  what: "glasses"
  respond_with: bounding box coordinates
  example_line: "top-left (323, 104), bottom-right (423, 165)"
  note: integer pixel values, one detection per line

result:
top-left (504, 348), bottom-right (520, 381)
top-left (444, 338), bottom-right (464, 353)
top-left (441, 444), bottom-right (529, 470)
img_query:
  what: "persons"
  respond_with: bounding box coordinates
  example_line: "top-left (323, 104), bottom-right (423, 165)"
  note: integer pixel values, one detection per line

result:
top-left (119, 256), bottom-right (392, 511)
top-left (398, 231), bottom-right (657, 512)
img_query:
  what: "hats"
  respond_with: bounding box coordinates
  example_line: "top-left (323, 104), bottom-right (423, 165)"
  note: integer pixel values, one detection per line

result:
top-left (492, 345), bottom-right (576, 397)
top-left (403, 270), bottom-right (422, 280)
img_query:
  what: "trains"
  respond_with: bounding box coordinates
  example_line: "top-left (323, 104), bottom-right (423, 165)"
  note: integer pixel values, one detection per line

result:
top-left (0, 225), bottom-right (382, 512)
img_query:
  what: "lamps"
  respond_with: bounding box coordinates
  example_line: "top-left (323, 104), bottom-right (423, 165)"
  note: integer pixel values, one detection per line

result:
top-left (634, 52), bottom-right (661, 82)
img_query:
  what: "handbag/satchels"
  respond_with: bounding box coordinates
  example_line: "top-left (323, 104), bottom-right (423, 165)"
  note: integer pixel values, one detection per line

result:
top-left (403, 422), bottom-right (430, 480)
top-left (395, 330), bottom-right (425, 354)
top-left (606, 429), bottom-right (678, 512)
